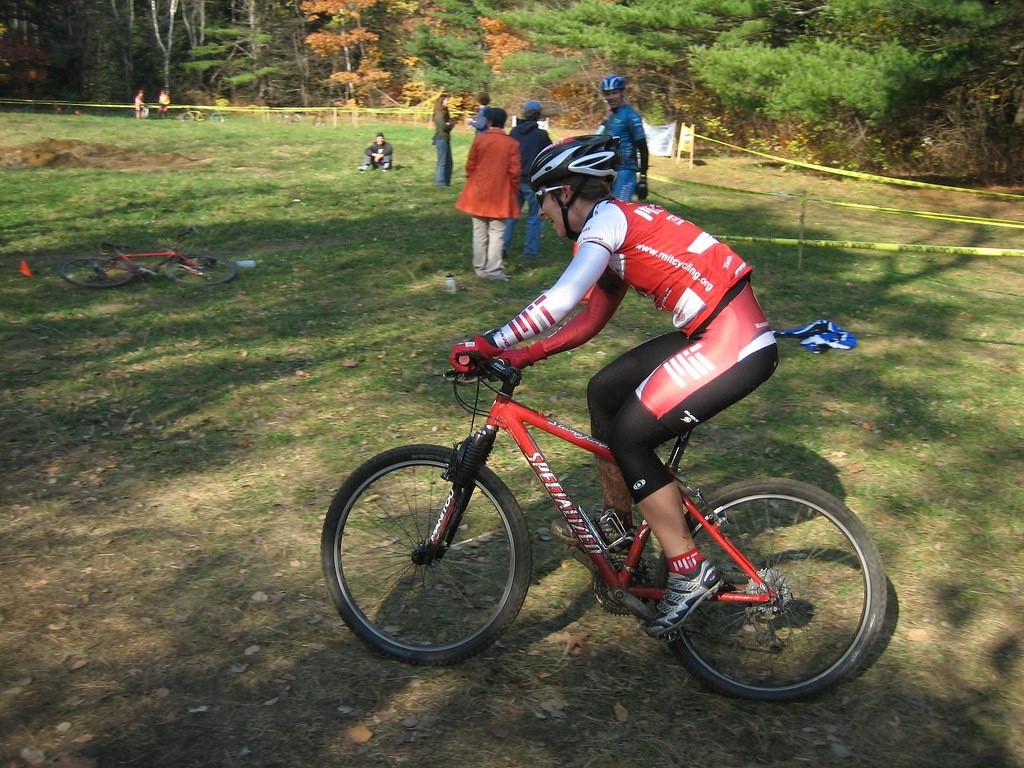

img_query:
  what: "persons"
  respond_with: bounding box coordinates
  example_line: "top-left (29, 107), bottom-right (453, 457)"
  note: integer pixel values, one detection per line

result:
top-left (451, 135), bottom-right (778, 635)
top-left (158, 90), bottom-right (170, 118)
top-left (454, 108), bottom-right (521, 279)
top-left (359, 132), bottom-right (392, 171)
top-left (432, 93), bottom-right (462, 187)
top-left (135, 90), bottom-right (145, 119)
top-left (467, 91), bottom-right (491, 135)
top-left (601, 75), bottom-right (649, 202)
top-left (502, 102), bottom-right (553, 267)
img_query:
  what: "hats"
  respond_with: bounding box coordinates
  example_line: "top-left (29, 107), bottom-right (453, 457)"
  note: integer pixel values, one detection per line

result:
top-left (525, 102), bottom-right (542, 112)
top-left (483, 108), bottom-right (507, 121)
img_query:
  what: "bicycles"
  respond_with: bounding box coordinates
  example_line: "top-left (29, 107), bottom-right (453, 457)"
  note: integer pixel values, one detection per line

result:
top-left (179, 105), bottom-right (328, 126)
top-left (57, 227), bottom-right (237, 289)
top-left (319, 349), bottom-right (888, 705)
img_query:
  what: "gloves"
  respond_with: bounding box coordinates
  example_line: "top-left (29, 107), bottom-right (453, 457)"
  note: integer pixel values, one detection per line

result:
top-left (451, 336), bottom-right (505, 373)
top-left (499, 340), bottom-right (547, 368)
top-left (635, 182), bottom-right (648, 200)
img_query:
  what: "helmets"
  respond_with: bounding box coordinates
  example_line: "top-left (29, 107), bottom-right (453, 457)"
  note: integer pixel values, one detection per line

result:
top-left (530, 135), bottom-right (622, 190)
top-left (601, 75), bottom-right (625, 90)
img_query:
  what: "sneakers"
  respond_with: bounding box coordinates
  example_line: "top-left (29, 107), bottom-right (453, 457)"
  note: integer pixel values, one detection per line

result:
top-left (642, 560), bottom-right (724, 638)
top-left (551, 518), bottom-right (638, 555)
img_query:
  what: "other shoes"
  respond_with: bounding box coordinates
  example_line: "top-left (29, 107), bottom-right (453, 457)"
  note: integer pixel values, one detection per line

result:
top-left (382, 163), bottom-right (390, 171)
top-left (524, 254), bottom-right (546, 259)
top-left (359, 165), bottom-right (369, 171)
top-left (503, 250), bottom-right (508, 259)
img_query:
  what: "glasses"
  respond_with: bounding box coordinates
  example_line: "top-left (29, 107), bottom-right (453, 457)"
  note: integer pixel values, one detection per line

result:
top-left (535, 185), bottom-right (572, 209)
